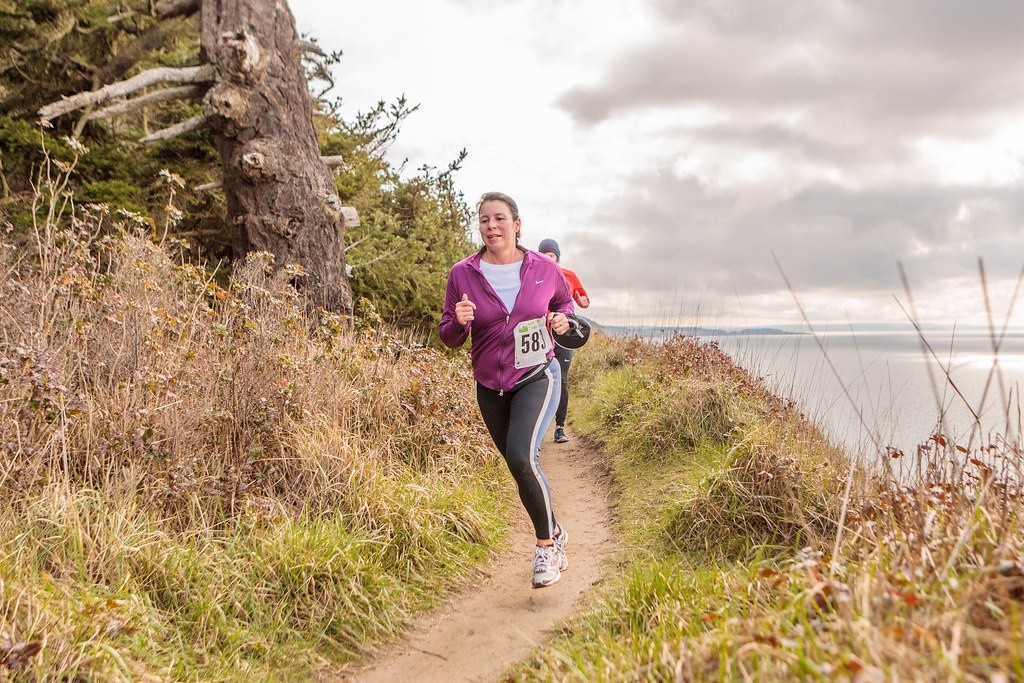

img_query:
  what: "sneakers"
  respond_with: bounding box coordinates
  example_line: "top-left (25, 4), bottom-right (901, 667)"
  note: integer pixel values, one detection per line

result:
top-left (532, 541), bottom-right (561, 587)
top-left (552, 522), bottom-right (568, 571)
top-left (554, 426), bottom-right (569, 443)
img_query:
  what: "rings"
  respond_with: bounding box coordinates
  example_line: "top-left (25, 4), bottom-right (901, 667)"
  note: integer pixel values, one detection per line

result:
top-left (559, 322), bottom-right (561, 326)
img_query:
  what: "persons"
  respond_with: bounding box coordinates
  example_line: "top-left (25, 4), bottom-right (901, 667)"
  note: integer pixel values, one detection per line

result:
top-left (437, 192), bottom-right (575, 588)
top-left (539, 239), bottom-right (590, 442)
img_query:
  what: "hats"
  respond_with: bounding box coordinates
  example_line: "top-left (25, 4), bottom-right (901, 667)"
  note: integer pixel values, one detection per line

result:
top-left (538, 239), bottom-right (560, 263)
